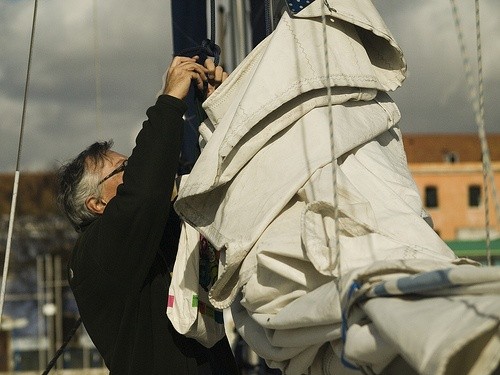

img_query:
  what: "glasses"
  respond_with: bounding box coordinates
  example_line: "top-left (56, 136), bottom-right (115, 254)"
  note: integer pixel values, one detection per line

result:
top-left (97, 160), bottom-right (130, 186)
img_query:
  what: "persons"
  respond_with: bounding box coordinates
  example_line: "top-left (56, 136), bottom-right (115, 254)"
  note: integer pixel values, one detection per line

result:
top-left (59, 54), bottom-right (233, 374)
